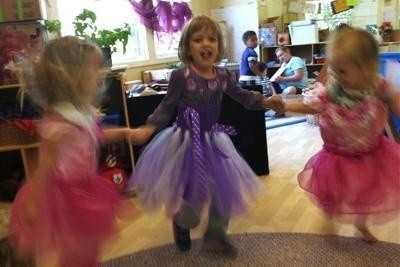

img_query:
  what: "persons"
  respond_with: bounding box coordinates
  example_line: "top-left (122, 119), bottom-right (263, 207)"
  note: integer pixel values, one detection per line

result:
top-left (271, 23), bottom-right (397, 243)
top-left (268, 45), bottom-right (309, 117)
top-left (256, 60), bottom-right (267, 76)
top-left (241, 30), bottom-right (265, 92)
top-left (7, 35), bottom-right (138, 267)
top-left (136, 15), bottom-right (287, 256)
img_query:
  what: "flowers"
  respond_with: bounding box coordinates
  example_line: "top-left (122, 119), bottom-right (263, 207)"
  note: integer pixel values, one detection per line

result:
top-left (378, 19), bottom-right (393, 36)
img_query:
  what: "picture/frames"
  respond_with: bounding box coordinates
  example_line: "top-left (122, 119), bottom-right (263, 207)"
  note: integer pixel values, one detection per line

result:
top-left (0, 18), bottom-right (52, 84)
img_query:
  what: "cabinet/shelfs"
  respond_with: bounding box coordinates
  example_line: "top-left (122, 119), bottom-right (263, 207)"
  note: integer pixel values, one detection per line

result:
top-left (1, 70), bottom-right (138, 204)
top-left (262, 40), bottom-right (328, 86)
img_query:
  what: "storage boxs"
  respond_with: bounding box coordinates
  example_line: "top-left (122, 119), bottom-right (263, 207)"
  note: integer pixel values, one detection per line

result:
top-left (288, 22), bottom-right (319, 44)
top-left (259, 27), bottom-right (277, 45)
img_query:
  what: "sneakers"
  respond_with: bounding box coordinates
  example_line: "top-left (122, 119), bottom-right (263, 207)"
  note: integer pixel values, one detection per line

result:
top-left (204, 230), bottom-right (236, 257)
top-left (173, 217), bottom-right (191, 251)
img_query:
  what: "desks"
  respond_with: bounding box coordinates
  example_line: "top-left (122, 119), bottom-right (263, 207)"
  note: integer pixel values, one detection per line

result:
top-left (215, 62), bottom-right (240, 83)
top-left (125, 83), bottom-right (270, 178)
top-left (378, 41), bottom-right (399, 52)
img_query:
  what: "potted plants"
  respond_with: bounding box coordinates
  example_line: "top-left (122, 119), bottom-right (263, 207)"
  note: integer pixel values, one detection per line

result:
top-left (42, 8), bottom-right (133, 69)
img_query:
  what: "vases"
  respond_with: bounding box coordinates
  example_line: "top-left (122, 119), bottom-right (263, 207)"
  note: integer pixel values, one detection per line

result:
top-left (382, 34), bottom-right (392, 42)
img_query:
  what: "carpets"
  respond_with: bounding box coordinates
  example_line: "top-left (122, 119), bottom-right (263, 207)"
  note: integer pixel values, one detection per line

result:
top-left (99, 230), bottom-right (400, 266)
